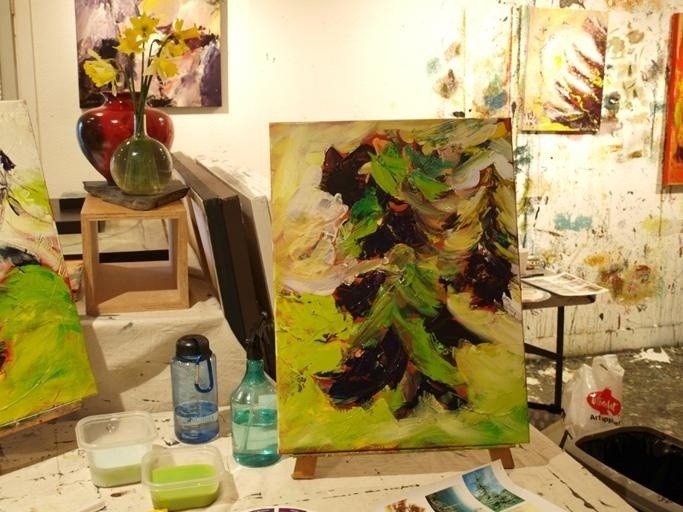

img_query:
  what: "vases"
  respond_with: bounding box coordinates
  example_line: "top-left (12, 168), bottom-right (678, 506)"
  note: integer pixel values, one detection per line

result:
top-left (76, 91), bottom-right (175, 186)
top-left (109, 114), bottom-right (174, 195)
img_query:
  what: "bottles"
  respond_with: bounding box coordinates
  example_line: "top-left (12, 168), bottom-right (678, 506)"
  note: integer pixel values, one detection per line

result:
top-left (170, 334), bottom-right (220, 444)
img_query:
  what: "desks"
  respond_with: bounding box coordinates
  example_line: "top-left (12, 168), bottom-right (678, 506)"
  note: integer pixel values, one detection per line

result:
top-left (80, 191), bottom-right (191, 315)
top-left (520, 264), bottom-right (596, 417)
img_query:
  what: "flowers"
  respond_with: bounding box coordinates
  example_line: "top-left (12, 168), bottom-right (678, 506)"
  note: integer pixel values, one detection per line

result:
top-left (83, 13), bottom-right (203, 114)
